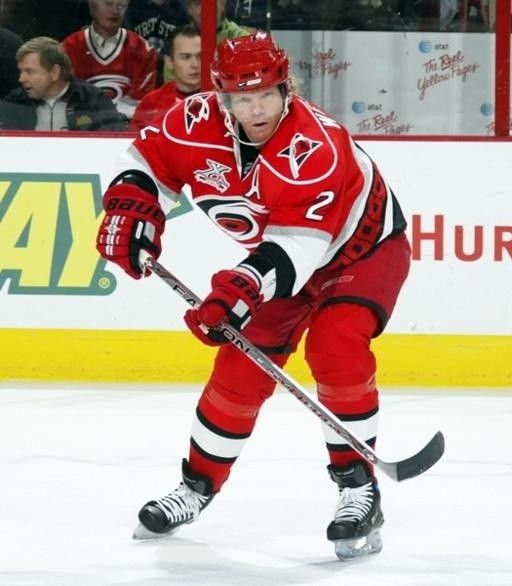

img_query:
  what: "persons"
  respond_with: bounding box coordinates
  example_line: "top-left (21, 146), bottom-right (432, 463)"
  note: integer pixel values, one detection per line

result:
top-left (0, 0), bottom-right (496, 137)
top-left (93, 31), bottom-right (413, 542)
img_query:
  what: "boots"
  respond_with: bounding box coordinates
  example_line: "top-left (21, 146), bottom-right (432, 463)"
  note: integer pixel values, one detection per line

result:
top-left (327, 462), bottom-right (385, 540)
top-left (139, 457), bottom-right (215, 532)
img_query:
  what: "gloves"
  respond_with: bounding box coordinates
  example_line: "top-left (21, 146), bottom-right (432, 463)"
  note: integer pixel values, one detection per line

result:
top-left (97, 183), bottom-right (165, 280)
top-left (184, 268), bottom-right (277, 346)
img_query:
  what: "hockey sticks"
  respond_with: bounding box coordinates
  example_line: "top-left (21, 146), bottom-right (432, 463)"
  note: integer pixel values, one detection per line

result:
top-left (139, 246), bottom-right (445, 482)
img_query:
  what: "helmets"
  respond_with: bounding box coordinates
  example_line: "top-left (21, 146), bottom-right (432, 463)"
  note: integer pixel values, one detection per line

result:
top-left (210, 29), bottom-right (293, 118)
